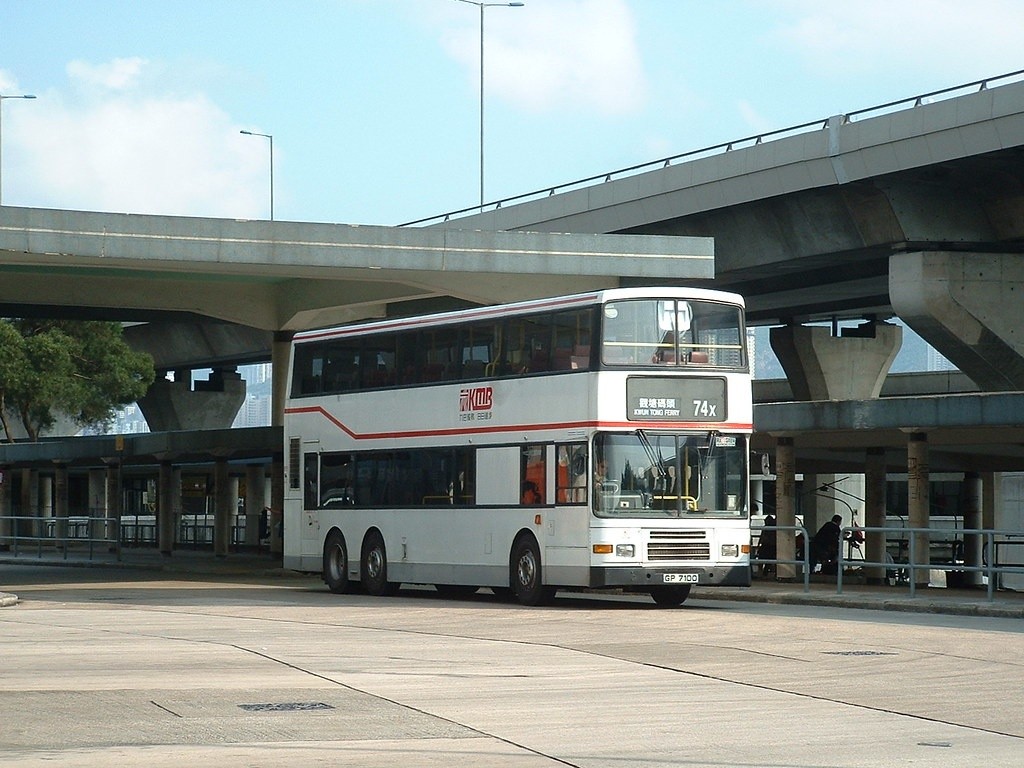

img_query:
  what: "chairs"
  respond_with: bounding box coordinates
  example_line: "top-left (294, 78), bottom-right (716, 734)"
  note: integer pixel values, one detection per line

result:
top-left (685, 351), bottom-right (712, 366)
top-left (304, 315), bottom-right (677, 391)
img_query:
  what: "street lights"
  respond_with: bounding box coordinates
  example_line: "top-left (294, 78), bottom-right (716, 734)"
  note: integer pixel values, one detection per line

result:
top-left (240, 130), bottom-right (274, 222)
top-left (455, 0), bottom-right (525, 212)
top-left (0, 93), bottom-right (38, 206)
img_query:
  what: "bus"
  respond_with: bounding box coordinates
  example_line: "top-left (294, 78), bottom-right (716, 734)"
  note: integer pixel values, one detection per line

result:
top-left (282, 287), bottom-right (771, 608)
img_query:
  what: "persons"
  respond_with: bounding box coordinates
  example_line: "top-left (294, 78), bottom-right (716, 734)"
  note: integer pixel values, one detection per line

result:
top-left (757, 515), bottom-right (776, 578)
top-left (750, 502), bottom-right (761, 578)
top-left (809, 515), bottom-right (849, 573)
top-left (572, 455), bottom-right (609, 511)
